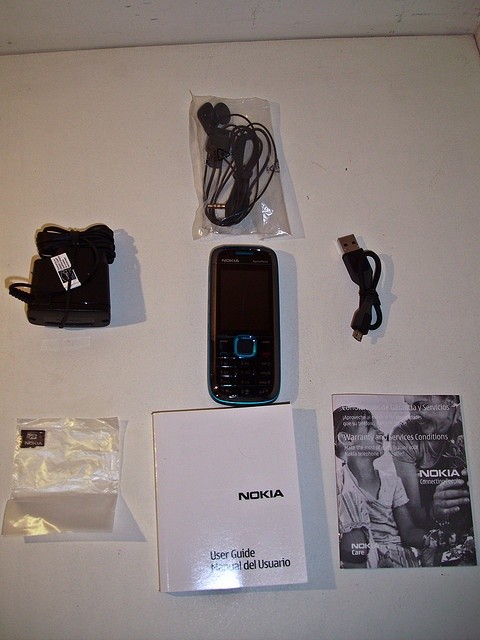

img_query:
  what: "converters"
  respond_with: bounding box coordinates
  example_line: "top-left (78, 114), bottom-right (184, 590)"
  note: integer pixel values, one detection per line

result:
top-left (7, 248), bottom-right (112, 330)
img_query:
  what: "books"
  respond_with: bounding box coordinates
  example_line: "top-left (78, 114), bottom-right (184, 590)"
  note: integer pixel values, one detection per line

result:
top-left (151, 403), bottom-right (310, 593)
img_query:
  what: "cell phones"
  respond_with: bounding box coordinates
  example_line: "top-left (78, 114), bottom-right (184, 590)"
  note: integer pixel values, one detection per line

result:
top-left (207, 245), bottom-right (282, 408)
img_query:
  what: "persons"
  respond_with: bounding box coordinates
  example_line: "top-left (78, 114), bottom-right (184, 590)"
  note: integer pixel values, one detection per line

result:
top-left (389, 394), bottom-right (478, 567)
top-left (332, 406), bottom-right (427, 569)
